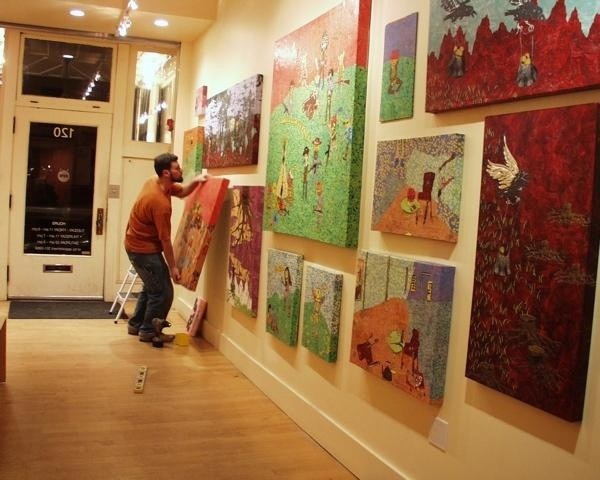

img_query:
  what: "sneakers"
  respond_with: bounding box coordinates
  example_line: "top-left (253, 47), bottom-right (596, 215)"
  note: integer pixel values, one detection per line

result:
top-left (127, 323), bottom-right (175, 343)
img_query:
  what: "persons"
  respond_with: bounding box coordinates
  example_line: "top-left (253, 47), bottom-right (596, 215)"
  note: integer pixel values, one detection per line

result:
top-left (124, 153), bottom-right (212, 343)
